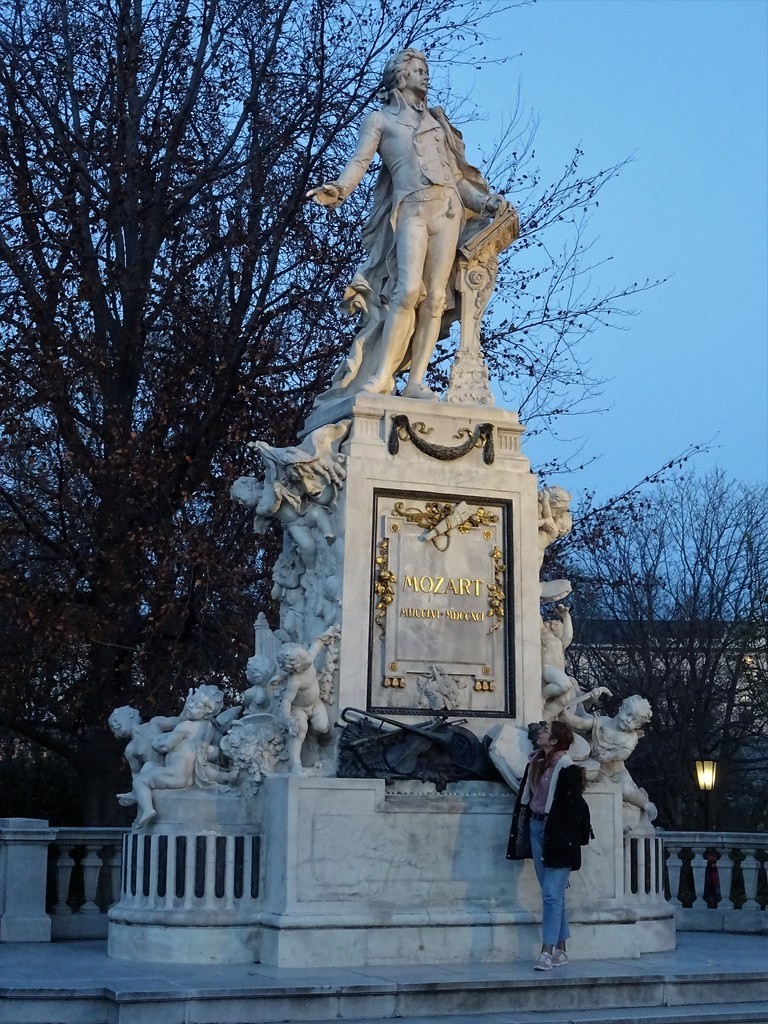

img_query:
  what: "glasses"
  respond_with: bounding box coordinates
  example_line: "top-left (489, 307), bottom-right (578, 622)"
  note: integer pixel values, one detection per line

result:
top-left (540, 726), bottom-right (553, 735)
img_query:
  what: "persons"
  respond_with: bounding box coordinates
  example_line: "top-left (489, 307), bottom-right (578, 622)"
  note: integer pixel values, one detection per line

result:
top-left (240, 655), bottom-right (275, 716)
top-left (506, 721), bottom-right (591, 970)
top-left (230, 443), bottom-right (336, 570)
top-left (307, 48), bottom-right (512, 398)
top-left (538, 487), bottom-right (658, 822)
top-left (278, 625), bottom-right (340, 774)
top-left (108, 685), bottom-right (239, 829)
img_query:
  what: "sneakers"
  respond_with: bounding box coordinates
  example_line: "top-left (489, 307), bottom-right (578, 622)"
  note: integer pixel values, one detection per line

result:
top-left (532, 952), bottom-right (554, 971)
top-left (552, 948), bottom-right (568, 966)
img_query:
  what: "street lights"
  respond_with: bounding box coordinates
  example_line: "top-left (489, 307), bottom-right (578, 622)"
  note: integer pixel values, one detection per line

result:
top-left (695, 750), bottom-right (719, 831)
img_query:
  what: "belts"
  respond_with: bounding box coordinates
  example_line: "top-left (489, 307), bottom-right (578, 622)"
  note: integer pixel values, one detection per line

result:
top-left (527, 810), bottom-right (545, 822)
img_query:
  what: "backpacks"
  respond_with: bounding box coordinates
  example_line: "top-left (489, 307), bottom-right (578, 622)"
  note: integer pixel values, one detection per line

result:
top-left (568, 785), bottom-right (595, 846)
